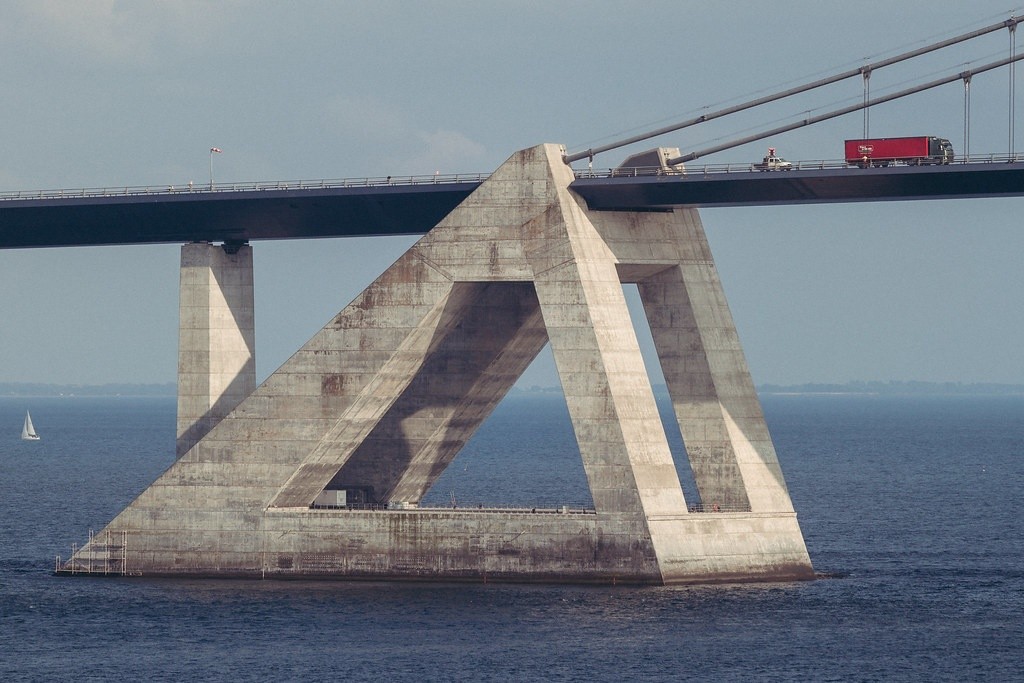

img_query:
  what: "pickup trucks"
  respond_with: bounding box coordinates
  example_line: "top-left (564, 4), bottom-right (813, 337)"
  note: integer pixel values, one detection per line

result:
top-left (753, 156), bottom-right (793, 172)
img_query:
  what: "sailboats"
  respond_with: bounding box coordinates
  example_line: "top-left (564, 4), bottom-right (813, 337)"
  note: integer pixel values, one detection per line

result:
top-left (19, 411), bottom-right (41, 441)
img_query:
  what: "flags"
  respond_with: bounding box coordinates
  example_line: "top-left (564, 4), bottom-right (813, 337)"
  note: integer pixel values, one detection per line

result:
top-left (211, 146), bottom-right (222, 153)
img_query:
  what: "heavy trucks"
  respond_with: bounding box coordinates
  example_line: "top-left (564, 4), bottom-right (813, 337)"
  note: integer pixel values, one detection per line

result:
top-left (843, 135), bottom-right (956, 169)
